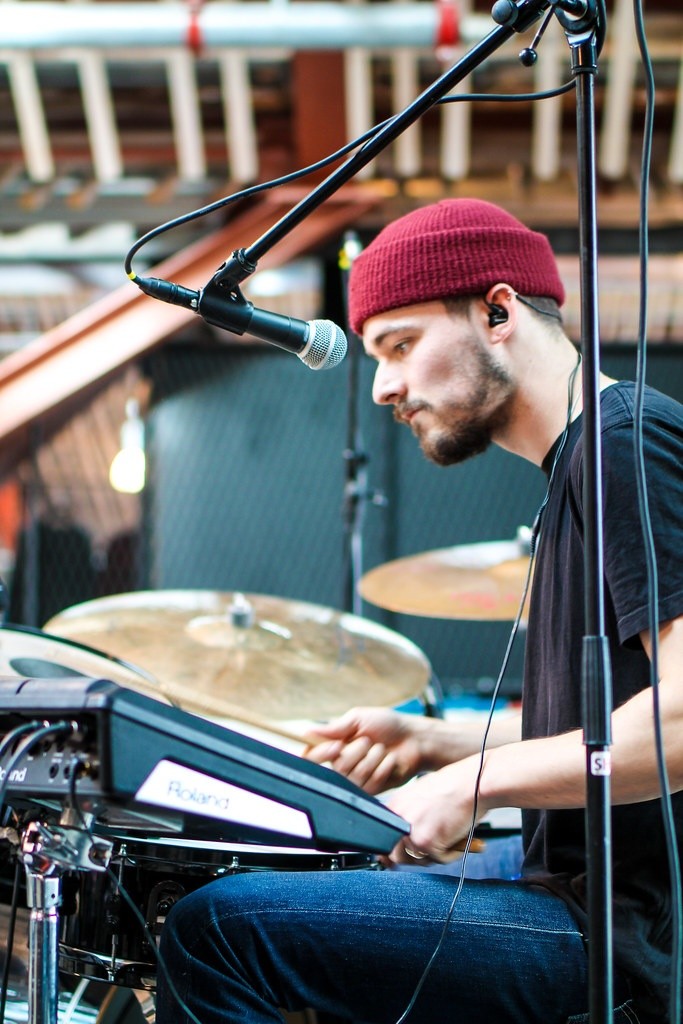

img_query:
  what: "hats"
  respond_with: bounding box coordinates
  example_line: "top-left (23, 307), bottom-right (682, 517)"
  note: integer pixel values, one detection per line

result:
top-left (346, 198), bottom-right (563, 336)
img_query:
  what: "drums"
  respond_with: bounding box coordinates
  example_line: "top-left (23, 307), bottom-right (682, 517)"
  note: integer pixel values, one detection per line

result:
top-left (0, 627), bottom-right (182, 712)
top-left (52, 835), bottom-right (384, 991)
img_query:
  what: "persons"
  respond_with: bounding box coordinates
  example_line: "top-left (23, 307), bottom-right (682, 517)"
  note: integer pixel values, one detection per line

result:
top-left (151, 195), bottom-right (683, 1023)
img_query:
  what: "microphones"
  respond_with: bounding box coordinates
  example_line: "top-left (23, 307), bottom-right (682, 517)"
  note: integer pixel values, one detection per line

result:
top-left (138, 278), bottom-right (348, 370)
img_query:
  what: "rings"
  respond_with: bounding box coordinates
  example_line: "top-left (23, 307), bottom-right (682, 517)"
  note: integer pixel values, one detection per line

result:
top-left (403, 844), bottom-right (428, 862)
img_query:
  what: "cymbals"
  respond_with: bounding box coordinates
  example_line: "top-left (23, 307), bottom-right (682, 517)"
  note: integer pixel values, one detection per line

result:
top-left (356, 538), bottom-right (536, 619)
top-left (43, 587), bottom-right (431, 727)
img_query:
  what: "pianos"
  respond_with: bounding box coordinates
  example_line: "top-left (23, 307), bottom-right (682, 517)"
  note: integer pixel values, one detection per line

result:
top-left (0, 674), bottom-right (415, 1023)
